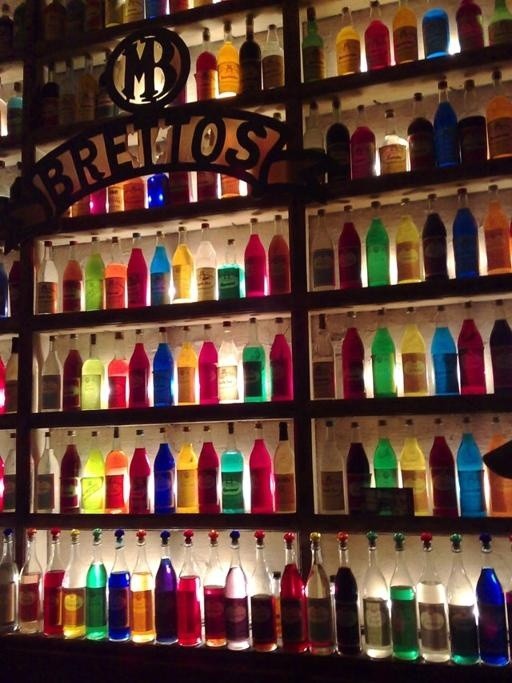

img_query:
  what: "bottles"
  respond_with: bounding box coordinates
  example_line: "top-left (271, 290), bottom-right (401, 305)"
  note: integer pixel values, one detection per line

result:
top-left (2, 314), bottom-right (291, 414)
top-left (4, 5), bottom-right (285, 142)
top-left (3, 420), bottom-right (295, 516)
top-left (11, 104), bottom-right (288, 220)
top-left (2, 211), bottom-right (289, 320)
top-left (310, 293), bottom-right (511, 400)
top-left (301, 57), bottom-right (511, 189)
top-left (301, 1), bottom-right (512, 86)
top-left (9, 530), bottom-right (510, 667)
top-left (309, 170), bottom-right (512, 294)
top-left (310, 418), bottom-right (512, 519)
top-left (3, 2), bottom-right (221, 35)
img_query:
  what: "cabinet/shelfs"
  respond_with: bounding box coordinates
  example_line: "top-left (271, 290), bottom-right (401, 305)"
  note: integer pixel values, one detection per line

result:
top-left (1, 0), bottom-right (512, 682)
